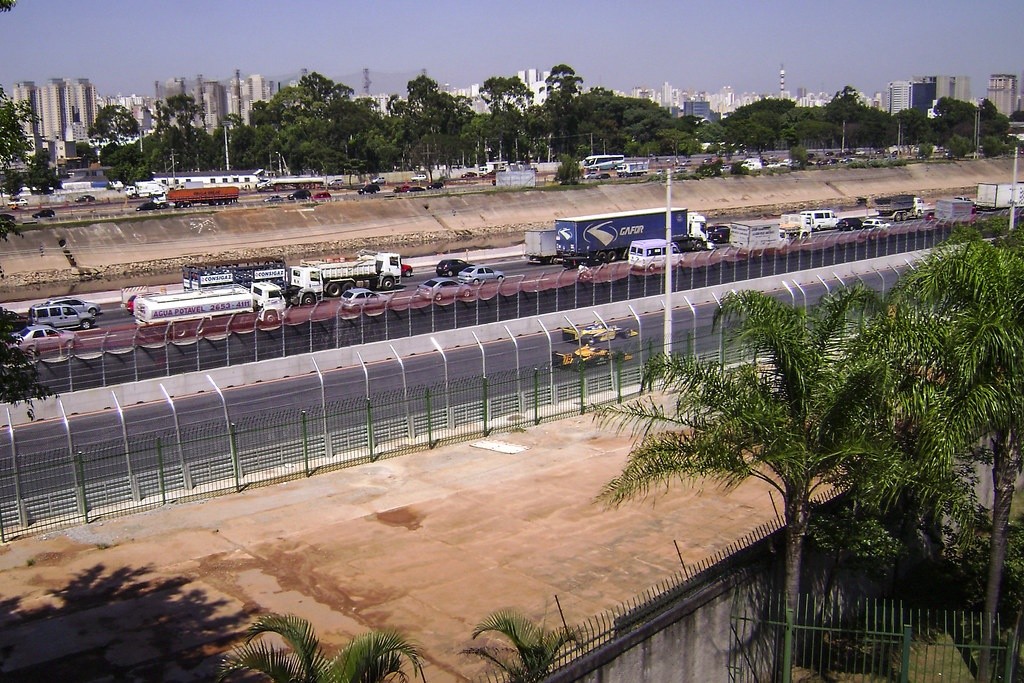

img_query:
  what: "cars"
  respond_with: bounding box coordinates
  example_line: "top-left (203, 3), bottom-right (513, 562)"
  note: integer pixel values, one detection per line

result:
top-left (560, 155), bottom-right (648, 185)
top-left (263, 189), bottom-right (331, 203)
top-left (0, 214), bottom-right (15, 222)
top-left (461, 161), bottom-right (508, 186)
top-left (75, 195), bottom-right (95, 202)
top-left (655, 158), bottom-right (793, 175)
top-left (821, 156), bottom-right (896, 165)
top-left (417, 259), bottom-right (505, 301)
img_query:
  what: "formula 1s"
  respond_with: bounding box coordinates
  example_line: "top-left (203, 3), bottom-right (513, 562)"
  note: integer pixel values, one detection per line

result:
top-left (562, 321), bottom-right (638, 346)
top-left (552, 344), bottom-right (632, 365)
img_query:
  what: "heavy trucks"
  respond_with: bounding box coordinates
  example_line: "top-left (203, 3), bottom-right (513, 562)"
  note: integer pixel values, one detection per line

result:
top-left (135, 186), bottom-right (239, 211)
top-left (255, 177), bottom-right (343, 192)
top-left (126, 247), bottom-right (413, 338)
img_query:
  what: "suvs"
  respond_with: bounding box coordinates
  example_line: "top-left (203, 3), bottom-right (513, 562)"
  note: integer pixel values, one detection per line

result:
top-left (32, 210), bottom-right (55, 219)
top-left (4, 297), bottom-right (101, 358)
top-left (357, 174), bottom-right (443, 194)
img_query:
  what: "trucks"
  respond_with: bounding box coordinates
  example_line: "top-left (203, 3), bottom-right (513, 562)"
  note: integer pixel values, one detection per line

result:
top-left (875, 182), bottom-right (1024, 224)
top-left (7, 199), bottom-right (28, 207)
top-left (124, 181), bottom-right (203, 199)
top-left (525, 207), bottom-right (891, 284)
